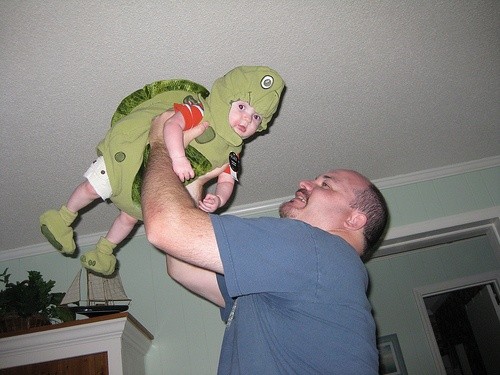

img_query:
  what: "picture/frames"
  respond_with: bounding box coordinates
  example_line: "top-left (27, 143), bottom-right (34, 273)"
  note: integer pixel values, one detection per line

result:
top-left (377, 334), bottom-right (409, 375)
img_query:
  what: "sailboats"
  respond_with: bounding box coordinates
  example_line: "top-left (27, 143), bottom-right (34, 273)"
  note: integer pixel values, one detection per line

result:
top-left (60, 267), bottom-right (133, 318)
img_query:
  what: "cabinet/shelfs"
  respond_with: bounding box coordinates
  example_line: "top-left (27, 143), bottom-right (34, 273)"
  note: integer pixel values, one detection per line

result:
top-left (0, 312), bottom-right (155, 375)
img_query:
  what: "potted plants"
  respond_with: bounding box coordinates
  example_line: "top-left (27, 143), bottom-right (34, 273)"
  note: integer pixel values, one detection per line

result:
top-left (0, 267), bottom-right (77, 335)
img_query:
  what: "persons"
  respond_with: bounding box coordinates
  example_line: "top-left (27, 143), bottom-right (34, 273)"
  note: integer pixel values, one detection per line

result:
top-left (140, 110), bottom-right (391, 374)
top-left (39, 65), bottom-right (285, 279)
